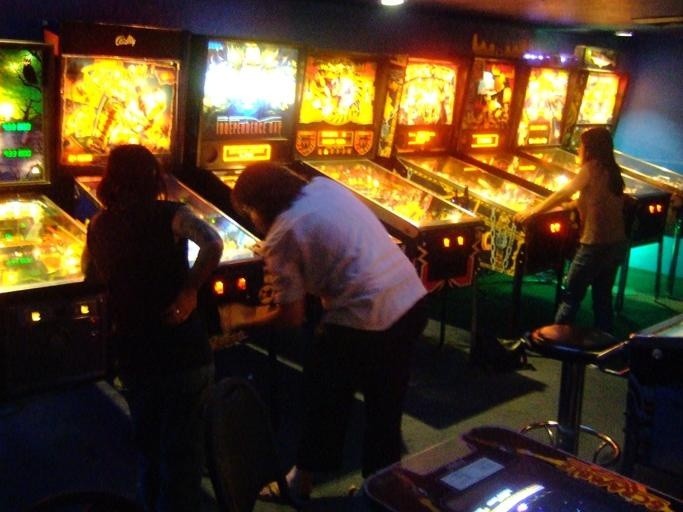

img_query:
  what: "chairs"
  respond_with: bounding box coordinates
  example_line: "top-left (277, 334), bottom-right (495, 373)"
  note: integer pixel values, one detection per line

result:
top-left (202, 372), bottom-right (383, 510)
top-left (27, 487), bottom-right (143, 512)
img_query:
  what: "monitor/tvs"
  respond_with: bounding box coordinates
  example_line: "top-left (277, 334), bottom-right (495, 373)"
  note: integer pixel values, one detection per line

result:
top-left (516, 66), bottom-right (571, 148)
top-left (294, 44), bottom-right (388, 160)
top-left (565, 67), bottom-right (620, 147)
top-left (59, 52), bottom-right (182, 168)
top-left (189, 36), bottom-right (307, 171)
top-left (0, 38), bottom-right (54, 188)
top-left (391, 52), bottom-right (463, 153)
top-left (461, 54), bottom-right (518, 151)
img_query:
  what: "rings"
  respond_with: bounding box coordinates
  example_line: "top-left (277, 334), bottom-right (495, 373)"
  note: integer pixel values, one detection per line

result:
top-left (175, 309), bottom-right (180, 314)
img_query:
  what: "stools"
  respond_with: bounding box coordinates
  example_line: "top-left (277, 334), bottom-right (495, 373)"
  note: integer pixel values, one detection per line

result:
top-left (520, 323), bottom-right (629, 468)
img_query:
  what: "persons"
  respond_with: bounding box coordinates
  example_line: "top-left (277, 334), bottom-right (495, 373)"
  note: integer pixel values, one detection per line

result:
top-left (513, 123), bottom-right (630, 346)
top-left (75, 142), bottom-right (224, 510)
top-left (229, 159), bottom-right (432, 500)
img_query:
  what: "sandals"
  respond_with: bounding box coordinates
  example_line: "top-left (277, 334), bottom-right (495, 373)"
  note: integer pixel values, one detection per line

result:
top-left (260, 481), bottom-right (309, 503)
